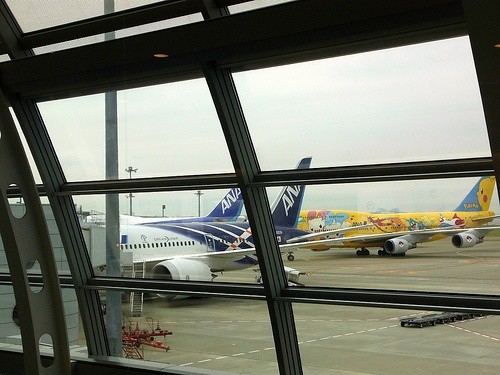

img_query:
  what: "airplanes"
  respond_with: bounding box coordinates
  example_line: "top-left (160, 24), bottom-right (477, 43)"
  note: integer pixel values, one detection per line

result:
top-left (297, 176), bottom-right (500, 258)
top-left (82, 156), bottom-right (466, 301)
